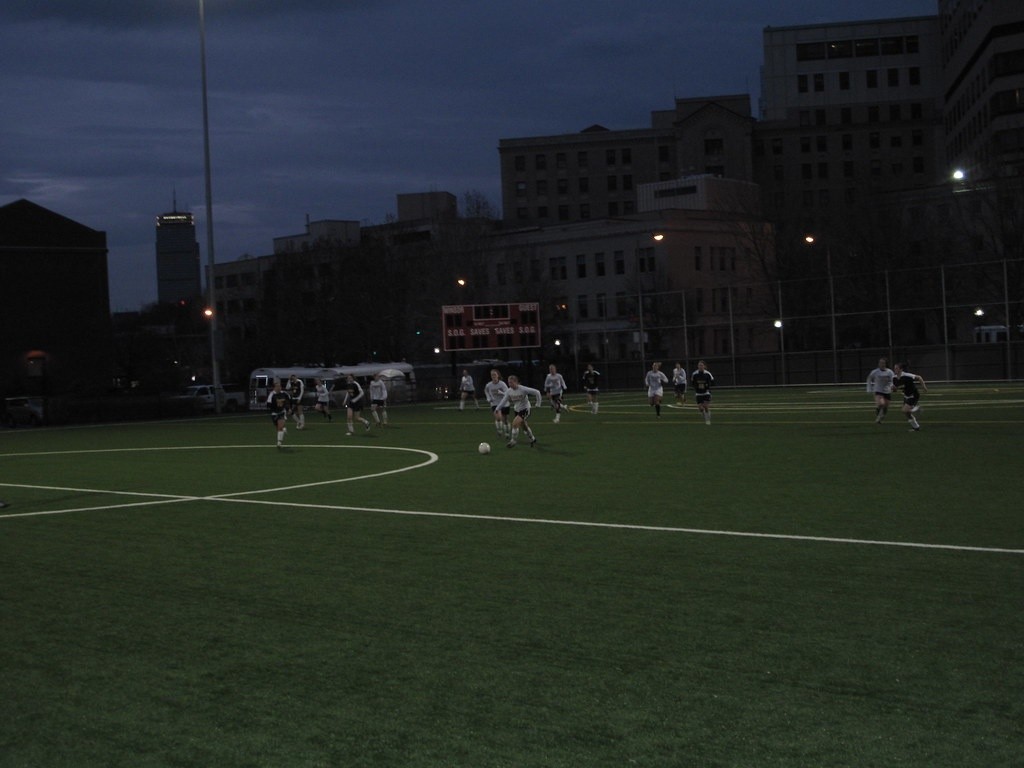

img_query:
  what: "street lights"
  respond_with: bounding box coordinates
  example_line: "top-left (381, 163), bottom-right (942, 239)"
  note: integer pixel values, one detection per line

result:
top-left (203, 306), bottom-right (221, 415)
top-left (635, 228), bottom-right (664, 388)
top-left (806, 234), bottom-right (837, 384)
top-left (952, 167), bottom-right (1012, 381)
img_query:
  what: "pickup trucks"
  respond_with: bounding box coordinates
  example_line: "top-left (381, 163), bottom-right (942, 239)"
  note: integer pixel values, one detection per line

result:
top-left (168, 385), bottom-right (246, 414)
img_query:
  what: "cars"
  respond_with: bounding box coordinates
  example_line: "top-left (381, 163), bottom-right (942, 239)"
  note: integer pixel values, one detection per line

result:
top-left (5, 397), bottom-right (70, 427)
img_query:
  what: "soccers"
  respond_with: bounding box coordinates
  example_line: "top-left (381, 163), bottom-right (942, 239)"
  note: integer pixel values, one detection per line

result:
top-left (478, 442), bottom-right (490, 454)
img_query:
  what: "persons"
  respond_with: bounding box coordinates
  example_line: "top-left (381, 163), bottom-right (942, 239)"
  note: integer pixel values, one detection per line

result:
top-left (646, 362), bottom-right (669, 418)
top-left (866, 357), bottom-right (896, 424)
top-left (285, 375), bottom-right (305, 429)
top-left (544, 365), bottom-right (568, 424)
top-left (484, 369), bottom-right (512, 440)
top-left (494, 375), bottom-right (542, 449)
top-left (315, 378), bottom-right (333, 422)
top-left (369, 374), bottom-right (388, 427)
top-left (672, 363), bottom-right (688, 406)
top-left (267, 382), bottom-right (292, 447)
top-left (342, 374), bottom-right (371, 435)
top-left (583, 364), bottom-right (601, 414)
top-left (890, 362), bottom-right (927, 431)
top-left (457, 369), bottom-right (479, 412)
top-left (691, 360), bottom-right (714, 424)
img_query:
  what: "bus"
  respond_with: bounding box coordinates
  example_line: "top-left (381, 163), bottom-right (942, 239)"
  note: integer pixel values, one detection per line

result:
top-left (249, 361), bottom-right (417, 411)
top-left (418, 359), bottom-right (545, 401)
